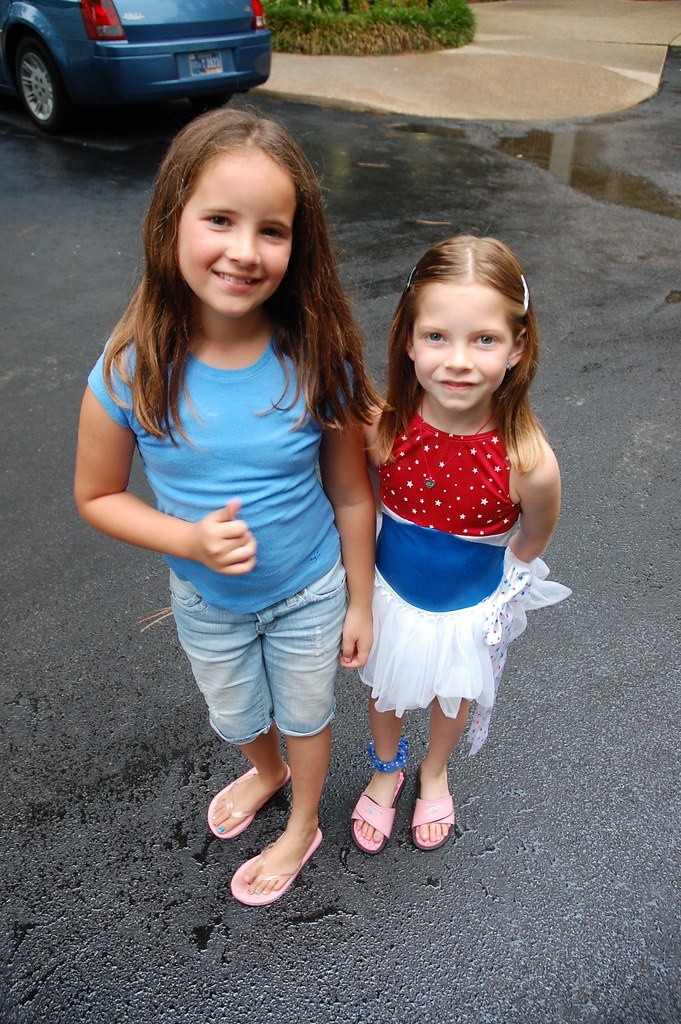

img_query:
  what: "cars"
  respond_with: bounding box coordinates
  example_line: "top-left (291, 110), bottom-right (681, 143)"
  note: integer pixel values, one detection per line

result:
top-left (0, 0), bottom-right (273, 137)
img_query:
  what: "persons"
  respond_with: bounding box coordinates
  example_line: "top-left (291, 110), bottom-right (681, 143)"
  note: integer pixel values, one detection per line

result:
top-left (73, 108), bottom-right (392, 906)
top-left (350, 236), bottom-right (572, 853)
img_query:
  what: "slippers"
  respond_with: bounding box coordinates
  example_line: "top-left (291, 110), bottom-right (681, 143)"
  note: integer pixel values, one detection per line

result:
top-left (351, 769), bottom-right (407, 855)
top-left (411, 765), bottom-right (456, 851)
top-left (208, 758), bottom-right (292, 840)
top-left (230, 827), bottom-right (323, 907)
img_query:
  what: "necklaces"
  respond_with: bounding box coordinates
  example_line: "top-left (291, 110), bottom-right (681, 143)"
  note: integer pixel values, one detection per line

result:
top-left (420, 393), bottom-right (495, 491)
